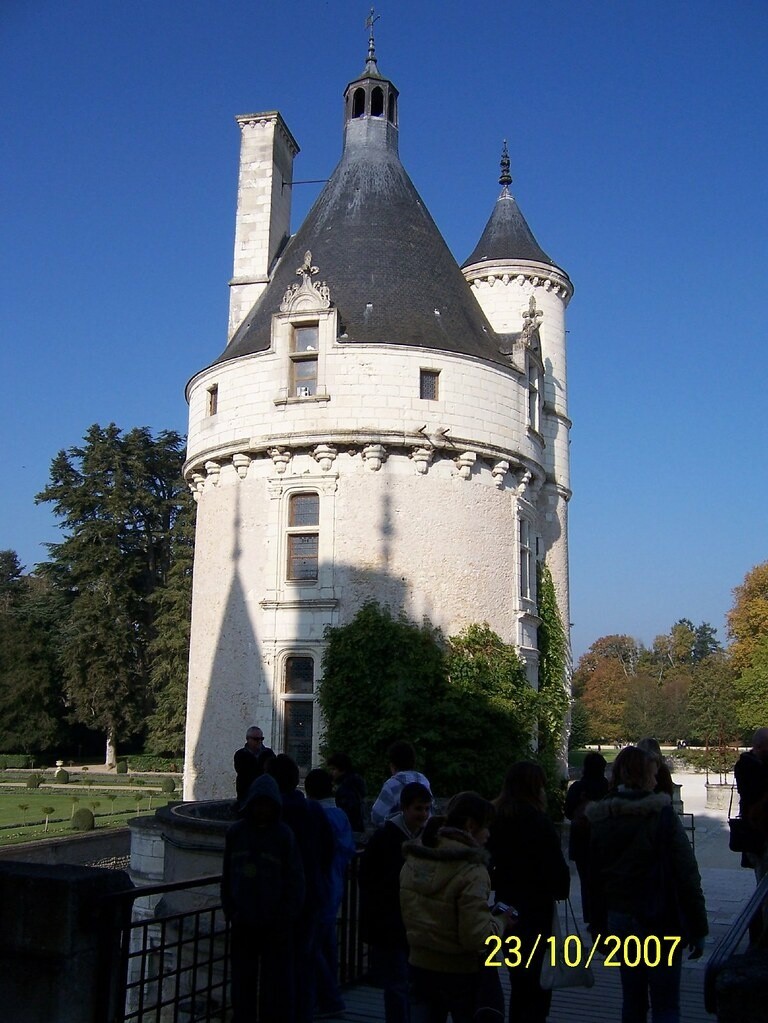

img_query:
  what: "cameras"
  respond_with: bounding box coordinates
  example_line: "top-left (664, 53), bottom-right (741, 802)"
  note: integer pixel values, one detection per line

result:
top-left (492, 902), bottom-right (518, 921)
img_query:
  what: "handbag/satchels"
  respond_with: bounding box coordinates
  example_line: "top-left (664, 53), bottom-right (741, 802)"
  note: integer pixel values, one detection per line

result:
top-left (729, 817), bottom-right (745, 852)
top-left (540, 897), bottom-right (595, 990)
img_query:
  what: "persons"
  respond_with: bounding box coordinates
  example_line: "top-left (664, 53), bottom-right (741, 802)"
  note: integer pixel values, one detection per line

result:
top-left (586, 747), bottom-right (708, 1023)
top-left (264, 754), bottom-right (335, 1023)
top-left (639, 738), bottom-right (674, 804)
top-left (357, 782), bottom-right (431, 1023)
top-left (221, 774), bottom-right (317, 1023)
top-left (484, 761), bottom-right (570, 1023)
top-left (327, 754), bottom-right (366, 832)
top-left (735, 728), bottom-right (768, 888)
top-left (305, 767), bottom-right (358, 1018)
top-left (399, 791), bottom-right (517, 1023)
top-left (563, 751), bottom-right (609, 923)
top-left (234, 727), bottom-right (276, 812)
top-left (370, 741), bottom-right (434, 824)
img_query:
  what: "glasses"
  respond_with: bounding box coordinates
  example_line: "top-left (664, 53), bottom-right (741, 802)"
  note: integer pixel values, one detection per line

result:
top-left (248, 736), bottom-right (264, 742)
